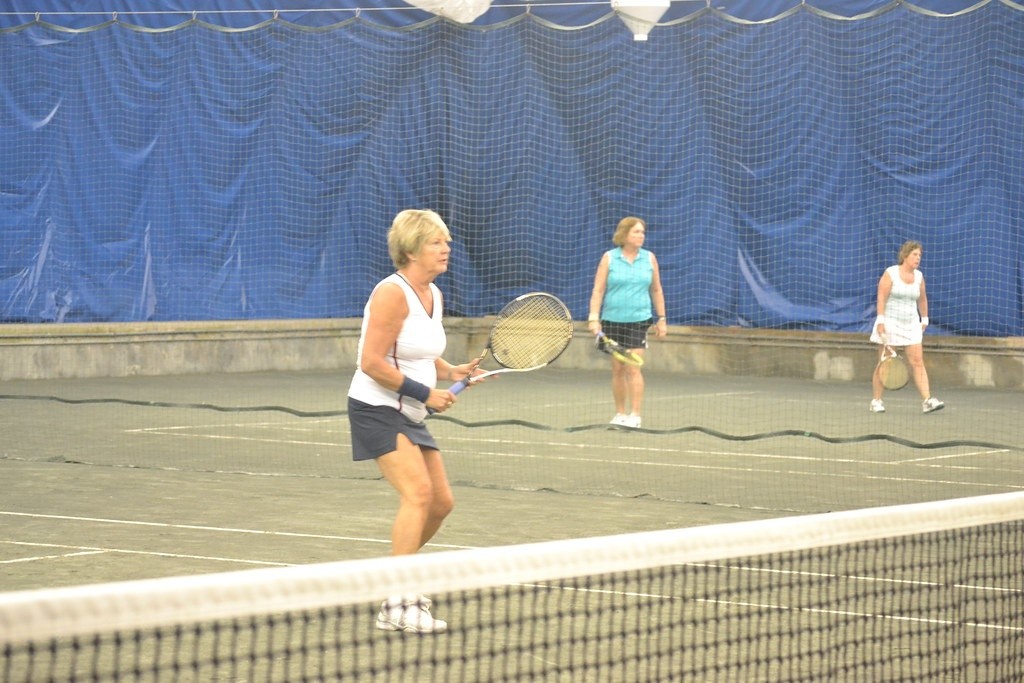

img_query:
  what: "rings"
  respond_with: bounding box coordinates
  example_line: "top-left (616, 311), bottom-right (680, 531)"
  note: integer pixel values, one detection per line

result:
top-left (448, 402), bottom-right (452, 404)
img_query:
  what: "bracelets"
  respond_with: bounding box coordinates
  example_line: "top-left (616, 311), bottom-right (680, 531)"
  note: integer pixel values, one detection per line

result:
top-left (877, 315), bottom-right (884, 325)
top-left (658, 316), bottom-right (666, 321)
top-left (588, 312), bottom-right (600, 322)
top-left (921, 316), bottom-right (929, 326)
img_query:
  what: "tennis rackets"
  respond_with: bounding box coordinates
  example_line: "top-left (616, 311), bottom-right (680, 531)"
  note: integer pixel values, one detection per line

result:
top-left (599, 332), bottom-right (643, 367)
top-left (424, 292), bottom-right (574, 415)
top-left (877, 333), bottom-right (913, 391)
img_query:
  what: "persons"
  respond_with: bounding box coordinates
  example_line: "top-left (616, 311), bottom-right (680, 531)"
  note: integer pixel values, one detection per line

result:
top-left (587, 217), bottom-right (668, 430)
top-left (346, 209), bottom-right (499, 634)
top-left (869, 240), bottom-right (944, 413)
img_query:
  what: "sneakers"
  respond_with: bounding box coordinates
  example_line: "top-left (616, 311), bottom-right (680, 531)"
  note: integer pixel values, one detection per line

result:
top-left (376, 600), bottom-right (448, 634)
top-left (609, 414), bottom-right (627, 427)
top-left (618, 415), bottom-right (643, 430)
top-left (869, 399), bottom-right (885, 413)
top-left (415, 595), bottom-right (434, 611)
top-left (922, 397), bottom-right (945, 413)
